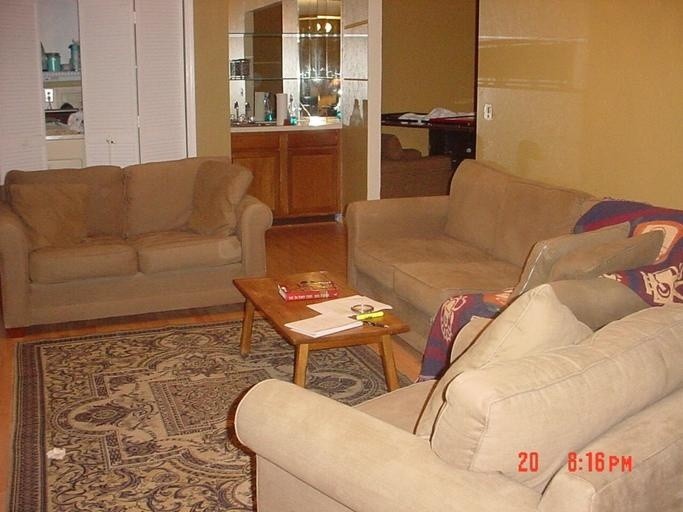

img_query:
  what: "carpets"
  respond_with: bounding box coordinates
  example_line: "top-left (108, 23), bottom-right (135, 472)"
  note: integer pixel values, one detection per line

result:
top-left (2, 312), bottom-right (421, 512)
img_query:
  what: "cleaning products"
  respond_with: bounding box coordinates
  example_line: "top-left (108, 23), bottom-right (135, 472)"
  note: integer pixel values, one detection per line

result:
top-left (263, 93), bottom-right (276, 120)
top-left (288, 92), bottom-right (300, 126)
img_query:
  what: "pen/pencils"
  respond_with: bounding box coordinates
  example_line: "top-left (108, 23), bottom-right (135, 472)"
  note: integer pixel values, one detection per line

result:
top-left (348, 311), bottom-right (390, 328)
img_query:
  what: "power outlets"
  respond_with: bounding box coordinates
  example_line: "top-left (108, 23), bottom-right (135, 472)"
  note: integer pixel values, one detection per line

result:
top-left (43, 88), bottom-right (54, 104)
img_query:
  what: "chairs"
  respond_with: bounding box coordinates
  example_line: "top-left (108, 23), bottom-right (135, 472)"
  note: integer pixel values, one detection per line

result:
top-left (380, 132), bottom-right (454, 197)
top-left (226, 302), bottom-right (681, 512)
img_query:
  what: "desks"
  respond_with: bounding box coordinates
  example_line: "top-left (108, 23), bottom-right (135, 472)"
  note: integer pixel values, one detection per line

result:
top-left (427, 112), bottom-right (475, 160)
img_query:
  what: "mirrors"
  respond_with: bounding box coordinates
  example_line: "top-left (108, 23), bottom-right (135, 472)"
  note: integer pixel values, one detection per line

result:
top-left (228, 0), bottom-right (342, 124)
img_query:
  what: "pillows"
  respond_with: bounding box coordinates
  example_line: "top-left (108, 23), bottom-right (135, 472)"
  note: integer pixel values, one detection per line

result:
top-left (4, 179), bottom-right (92, 252)
top-left (383, 134), bottom-right (405, 160)
top-left (412, 280), bottom-right (597, 433)
top-left (179, 158), bottom-right (254, 240)
top-left (507, 220), bottom-right (631, 299)
top-left (549, 228), bottom-right (666, 282)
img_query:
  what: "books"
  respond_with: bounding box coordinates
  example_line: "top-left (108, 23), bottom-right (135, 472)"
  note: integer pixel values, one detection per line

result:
top-left (284, 311), bottom-right (364, 339)
top-left (277, 280), bottom-right (339, 301)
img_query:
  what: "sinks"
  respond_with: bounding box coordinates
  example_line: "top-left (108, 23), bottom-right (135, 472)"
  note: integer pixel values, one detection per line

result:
top-left (232, 122), bottom-right (268, 128)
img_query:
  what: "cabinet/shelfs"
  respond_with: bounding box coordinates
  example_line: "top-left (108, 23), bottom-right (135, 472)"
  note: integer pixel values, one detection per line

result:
top-left (231, 131), bottom-right (341, 221)
top-left (0, 0), bottom-right (196, 185)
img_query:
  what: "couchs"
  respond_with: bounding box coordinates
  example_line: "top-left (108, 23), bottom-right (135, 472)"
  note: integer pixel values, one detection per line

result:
top-left (337, 156), bottom-right (681, 363)
top-left (0, 152), bottom-right (274, 333)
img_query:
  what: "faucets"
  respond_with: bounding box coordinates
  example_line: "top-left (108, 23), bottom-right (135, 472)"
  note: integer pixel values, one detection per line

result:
top-left (244, 102), bottom-right (252, 124)
top-left (233, 100), bottom-right (241, 123)
